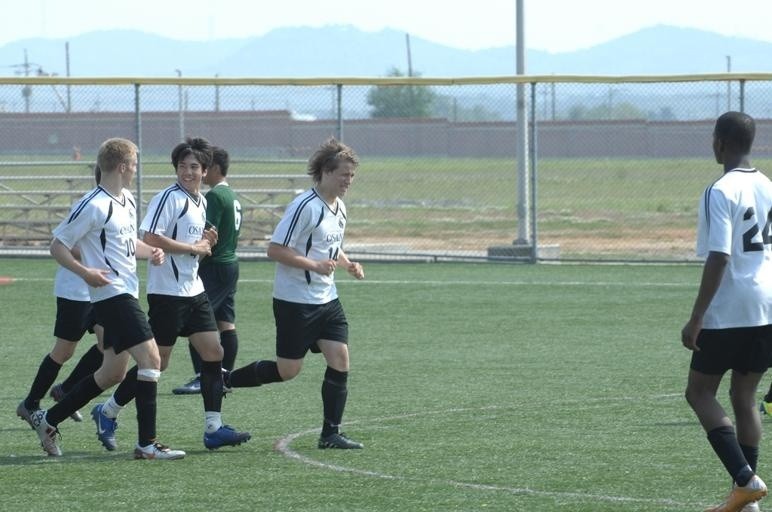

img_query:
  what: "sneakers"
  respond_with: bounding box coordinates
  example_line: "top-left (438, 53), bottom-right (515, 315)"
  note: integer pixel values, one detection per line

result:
top-left (91, 403), bottom-right (119, 451)
top-left (220, 367), bottom-right (233, 399)
top-left (318, 431), bottom-right (364, 449)
top-left (134, 440), bottom-right (186, 460)
top-left (16, 401), bottom-right (63, 457)
top-left (50, 384), bottom-right (83, 421)
top-left (172, 373), bottom-right (202, 394)
top-left (704, 474), bottom-right (768, 512)
top-left (759, 397), bottom-right (772, 418)
top-left (203, 424), bottom-right (251, 449)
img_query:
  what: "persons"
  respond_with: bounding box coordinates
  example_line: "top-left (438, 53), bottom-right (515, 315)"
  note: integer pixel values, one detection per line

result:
top-left (17, 164), bottom-right (105, 430)
top-left (29, 137), bottom-right (186, 461)
top-left (221, 137), bottom-right (365, 449)
top-left (682, 112), bottom-right (772, 512)
top-left (90, 137), bottom-right (252, 452)
top-left (759, 381), bottom-right (772, 418)
top-left (171, 144), bottom-right (244, 395)
